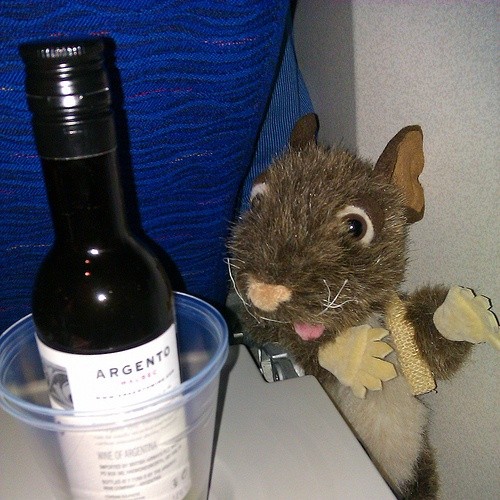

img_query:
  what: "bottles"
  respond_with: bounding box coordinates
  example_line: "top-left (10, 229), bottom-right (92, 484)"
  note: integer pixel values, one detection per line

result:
top-left (20, 36), bottom-right (193, 500)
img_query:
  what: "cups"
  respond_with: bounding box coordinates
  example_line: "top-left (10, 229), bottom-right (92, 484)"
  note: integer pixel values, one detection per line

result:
top-left (0, 290), bottom-right (229, 500)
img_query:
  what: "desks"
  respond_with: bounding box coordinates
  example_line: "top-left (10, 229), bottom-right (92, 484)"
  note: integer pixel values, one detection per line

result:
top-left (0, 344), bottom-right (397, 500)
